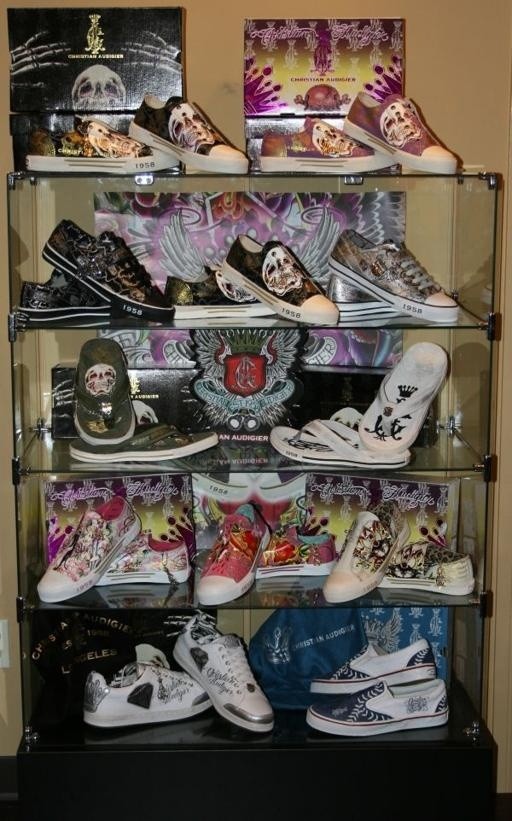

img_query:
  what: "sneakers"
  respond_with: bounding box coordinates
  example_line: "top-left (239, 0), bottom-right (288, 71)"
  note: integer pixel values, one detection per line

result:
top-left (40, 220), bottom-right (176, 324)
top-left (304, 638), bottom-right (450, 736)
top-left (129, 95), bottom-right (250, 174)
top-left (13, 266), bottom-right (141, 323)
top-left (323, 499), bottom-right (409, 603)
top-left (255, 523), bottom-right (339, 579)
top-left (83, 658), bottom-right (211, 728)
top-left (221, 233), bottom-right (339, 326)
top-left (344, 92), bottom-right (460, 178)
top-left (23, 121), bottom-right (180, 176)
top-left (376, 539), bottom-right (475, 595)
top-left (174, 611), bottom-right (277, 732)
top-left (94, 530), bottom-right (191, 585)
top-left (328, 228), bottom-right (460, 323)
top-left (37, 496), bottom-right (142, 603)
top-left (164, 265), bottom-right (278, 320)
top-left (197, 504), bottom-right (270, 605)
top-left (248, 120), bottom-right (397, 177)
top-left (326, 274), bottom-right (413, 322)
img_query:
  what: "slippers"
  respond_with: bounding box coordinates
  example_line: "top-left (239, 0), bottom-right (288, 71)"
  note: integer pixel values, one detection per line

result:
top-left (268, 342), bottom-right (450, 470)
top-left (68, 339), bottom-right (221, 464)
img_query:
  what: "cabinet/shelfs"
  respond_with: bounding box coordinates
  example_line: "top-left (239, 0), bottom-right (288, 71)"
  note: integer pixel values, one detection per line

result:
top-left (4, 166), bottom-right (500, 818)
